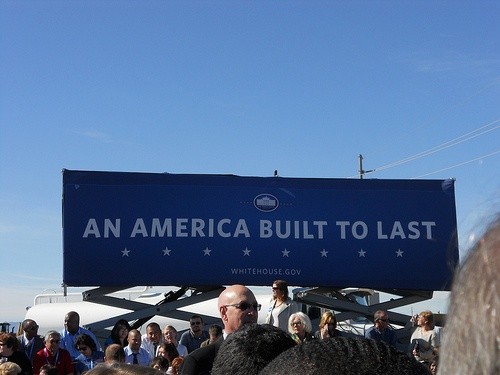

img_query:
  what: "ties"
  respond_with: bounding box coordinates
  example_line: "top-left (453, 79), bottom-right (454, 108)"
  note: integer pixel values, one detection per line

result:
top-left (27, 343), bottom-right (31, 351)
top-left (133, 353), bottom-right (138, 365)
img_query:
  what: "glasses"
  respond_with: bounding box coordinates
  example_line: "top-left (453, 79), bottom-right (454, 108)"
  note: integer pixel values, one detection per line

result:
top-left (224, 304), bottom-right (261, 311)
top-left (47, 340), bottom-right (60, 344)
top-left (77, 346), bottom-right (89, 351)
top-left (328, 323), bottom-right (335, 326)
top-left (191, 322), bottom-right (201, 325)
top-left (291, 322), bottom-right (302, 326)
top-left (273, 287), bottom-right (278, 290)
top-left (378, 319), bottom-right (388, 323)
top-left (25, 325), bottom-right (39, 332)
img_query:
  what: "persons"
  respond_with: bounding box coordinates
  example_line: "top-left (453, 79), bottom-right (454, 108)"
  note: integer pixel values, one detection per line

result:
top-left (179, 284), bottom-right (261, 375)
top-left (265, 280), bottom-right (298, 333)
top-left (0, 207), bottom-right (500, 375)
